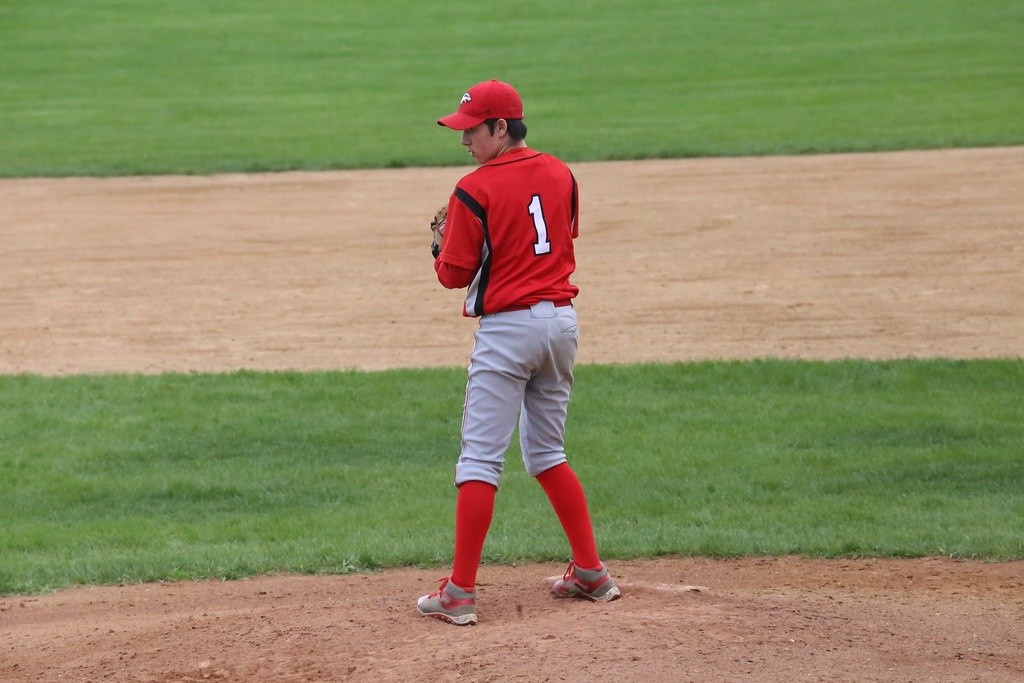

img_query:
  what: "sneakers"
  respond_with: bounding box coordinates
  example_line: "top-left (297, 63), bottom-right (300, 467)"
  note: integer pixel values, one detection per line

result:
top-left (416, 577), bottom-right (479, 625)
top-left (553, 560), bottom-right (620, 603)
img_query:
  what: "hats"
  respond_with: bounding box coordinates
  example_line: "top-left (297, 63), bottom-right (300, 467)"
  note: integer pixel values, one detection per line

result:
top-left (437, 79), bottom-right (524, 130)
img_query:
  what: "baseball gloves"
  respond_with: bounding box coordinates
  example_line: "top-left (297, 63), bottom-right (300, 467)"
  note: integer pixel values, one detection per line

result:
top-left (431, 202), bottom-right (448, 255)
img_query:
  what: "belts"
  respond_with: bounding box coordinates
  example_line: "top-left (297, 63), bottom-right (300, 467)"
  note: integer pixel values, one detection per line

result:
top-left (498, 298), bottom-right (571, 312)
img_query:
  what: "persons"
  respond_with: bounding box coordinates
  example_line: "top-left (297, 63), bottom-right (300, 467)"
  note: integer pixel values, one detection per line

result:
top-left (417, 79), bottom-right (621, 626)
